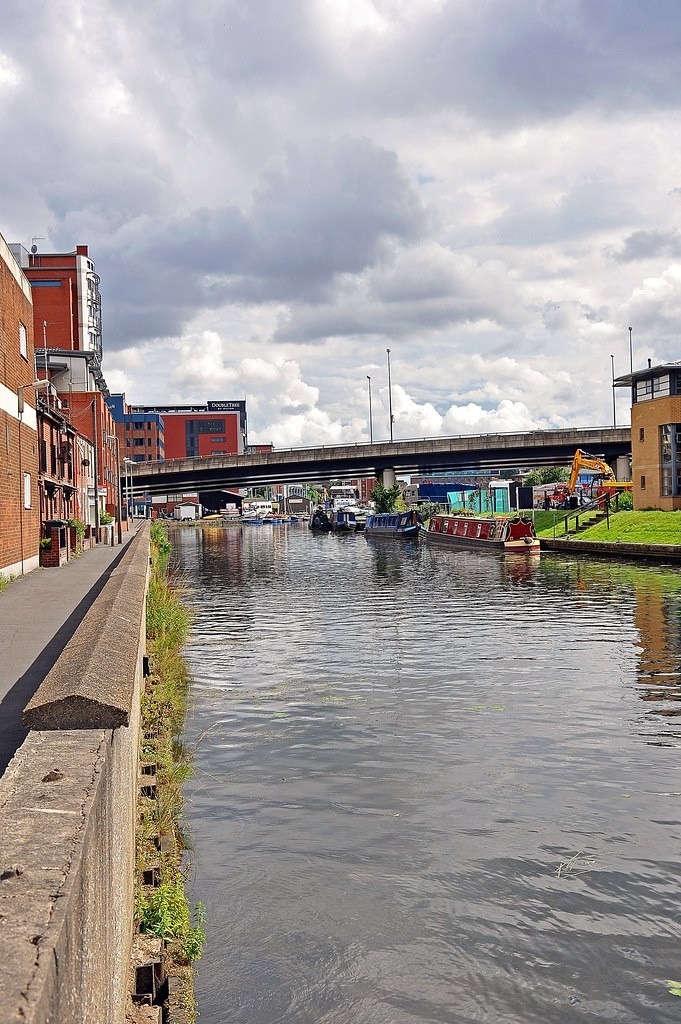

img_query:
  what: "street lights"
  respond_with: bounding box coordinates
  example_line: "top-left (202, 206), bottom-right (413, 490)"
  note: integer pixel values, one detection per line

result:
top-left (628, 327), bottom-right (633, 374)
top-left (367, 376), bottom-right (372, 445)
top-left (610, 354), bottom-right (616, 430)
top-left (130, 462), bottom-right (137, 523)
top-left (386, 348), bottom-right (392, 443)
top-left (123, 456), bottom-right (132, 532)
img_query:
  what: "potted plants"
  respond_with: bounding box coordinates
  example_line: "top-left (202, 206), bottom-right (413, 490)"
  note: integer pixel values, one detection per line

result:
top-left (39, 538), bottom-right (52, 551)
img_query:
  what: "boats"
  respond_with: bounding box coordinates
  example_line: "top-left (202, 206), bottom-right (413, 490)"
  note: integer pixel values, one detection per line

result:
top-left (241, 511), bottom-right (311, 525)
top-left (308, 506), bottom-right (375, 533)
top-left (418, 510), bottom-right (541, 551)
top-left (363, 508), bottom-right (423, 536)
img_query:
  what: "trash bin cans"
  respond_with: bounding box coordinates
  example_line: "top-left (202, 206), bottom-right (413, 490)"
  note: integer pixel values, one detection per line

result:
top-left (42, 519), bottom-right (68, 549)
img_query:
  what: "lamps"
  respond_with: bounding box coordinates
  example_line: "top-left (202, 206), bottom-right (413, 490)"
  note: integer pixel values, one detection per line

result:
top-left (20, 380), bottom-right (51, 390)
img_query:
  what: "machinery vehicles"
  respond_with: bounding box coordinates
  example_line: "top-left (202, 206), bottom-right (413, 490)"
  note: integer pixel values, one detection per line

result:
top-left (566, 449), bottom-right (633, 495)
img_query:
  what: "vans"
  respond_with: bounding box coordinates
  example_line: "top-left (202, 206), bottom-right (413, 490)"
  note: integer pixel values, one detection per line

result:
top-left (533, 482), bottom-right (599, 508)
